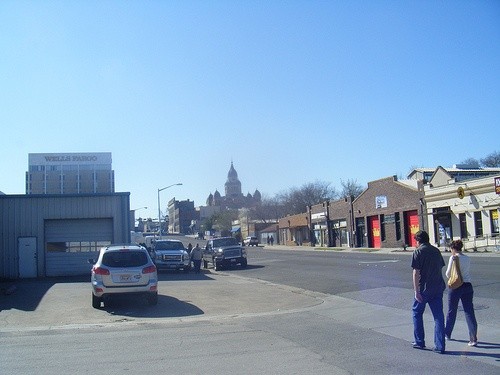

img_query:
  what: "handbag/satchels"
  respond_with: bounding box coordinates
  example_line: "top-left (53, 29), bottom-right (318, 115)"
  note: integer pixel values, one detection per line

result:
top-left (447, 256), bottom-right (464, 290)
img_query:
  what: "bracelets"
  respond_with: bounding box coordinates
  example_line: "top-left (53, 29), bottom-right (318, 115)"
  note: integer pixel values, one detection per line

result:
top-left (415, 291), bottom-right (420, 294)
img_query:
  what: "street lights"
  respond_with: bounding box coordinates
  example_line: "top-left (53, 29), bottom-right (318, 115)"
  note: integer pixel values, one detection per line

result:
top-left (134, 206), bottom-right (148, 211)
top-left (157, 182), bottom-right (183, 239)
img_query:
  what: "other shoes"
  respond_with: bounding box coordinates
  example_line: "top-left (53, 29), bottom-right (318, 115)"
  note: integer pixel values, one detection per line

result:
top-left (445, 336), bottom-right (451, 339)
top-left (410, 342), bottom-right (425, 349)
top-left (467, 340), bottom-right (477, 346)
top-left (432, 346), bottom-right (445, 354)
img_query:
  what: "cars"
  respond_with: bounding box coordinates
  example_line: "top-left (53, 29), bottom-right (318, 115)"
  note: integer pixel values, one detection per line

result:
top-left (194, 232), bottom-right (204, 240)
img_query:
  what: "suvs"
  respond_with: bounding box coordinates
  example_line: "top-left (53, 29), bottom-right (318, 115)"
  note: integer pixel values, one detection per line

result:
top-left (202, 236), bottom-right (248, 271)
top-left (243, 236), bottom-right (259, 247)
top-left (87, 243), bottom-right (159, 309)
top-left (150, 239), bottom-right (193, 272)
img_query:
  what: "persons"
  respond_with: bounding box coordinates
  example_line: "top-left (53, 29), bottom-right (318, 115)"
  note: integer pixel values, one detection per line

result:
top-left (411, 230), bottom-right (445, 354)
top-left (187, 243), bottom-right (208, 273)
top-left (445, 240), bottom-right (478, 346)
top-left (267, 235), bottom-right (274, 246)
